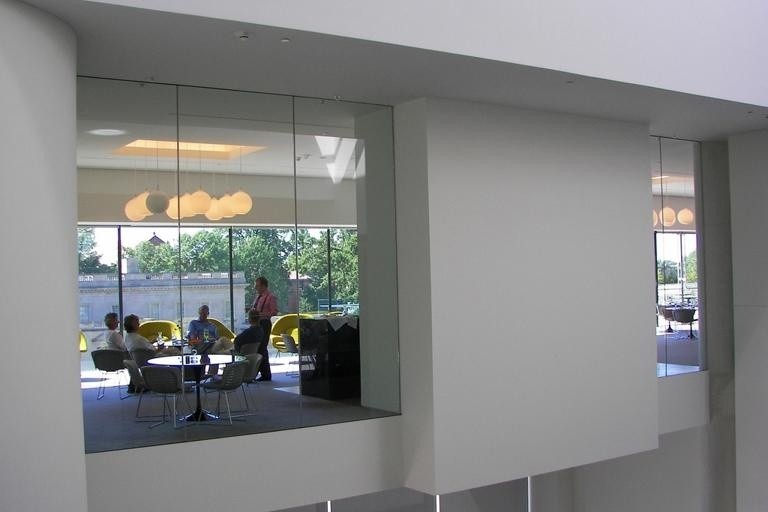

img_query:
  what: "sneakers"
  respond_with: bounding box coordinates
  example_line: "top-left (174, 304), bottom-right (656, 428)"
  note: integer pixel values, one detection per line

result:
top-left (127, 385), bottom-right (149, 392)
top-left (257, 376), bottom-right (271, 382)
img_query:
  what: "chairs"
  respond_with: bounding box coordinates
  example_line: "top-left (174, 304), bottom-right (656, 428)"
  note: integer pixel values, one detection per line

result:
top-left (80, 332), bottom-right (88, 352)
top-left (91, 318), bottom-right (263, 429)
top-left (271, 311), bottom-right (343, 358)
top-left (658, 297), bottom-right (698, 342)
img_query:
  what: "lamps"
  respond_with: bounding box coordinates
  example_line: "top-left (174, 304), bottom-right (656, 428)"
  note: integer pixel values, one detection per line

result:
top-left (652, 184), bottom-right (695, 228)
top-left (110, 139), bottom-right (272, 222)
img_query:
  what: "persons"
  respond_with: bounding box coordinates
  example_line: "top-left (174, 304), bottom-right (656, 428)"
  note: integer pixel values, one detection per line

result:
top-left (244, 277), bottom-right (277, 385)
top-left (186, 305), bottom-right (219, 379)
top-left (103, 312), bottom-right (149, 394)
top-left (123, 313), bottom-right (196, 393)
top-left (203, 308), bottom-right (263, 383)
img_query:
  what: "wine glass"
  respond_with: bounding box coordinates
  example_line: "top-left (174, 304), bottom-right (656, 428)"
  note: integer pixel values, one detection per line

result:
top-left (204, 330), bottom-right (209, 343)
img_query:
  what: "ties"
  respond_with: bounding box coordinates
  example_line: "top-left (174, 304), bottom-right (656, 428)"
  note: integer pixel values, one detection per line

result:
top-left (254, 295), bottom-right (261, 308)
